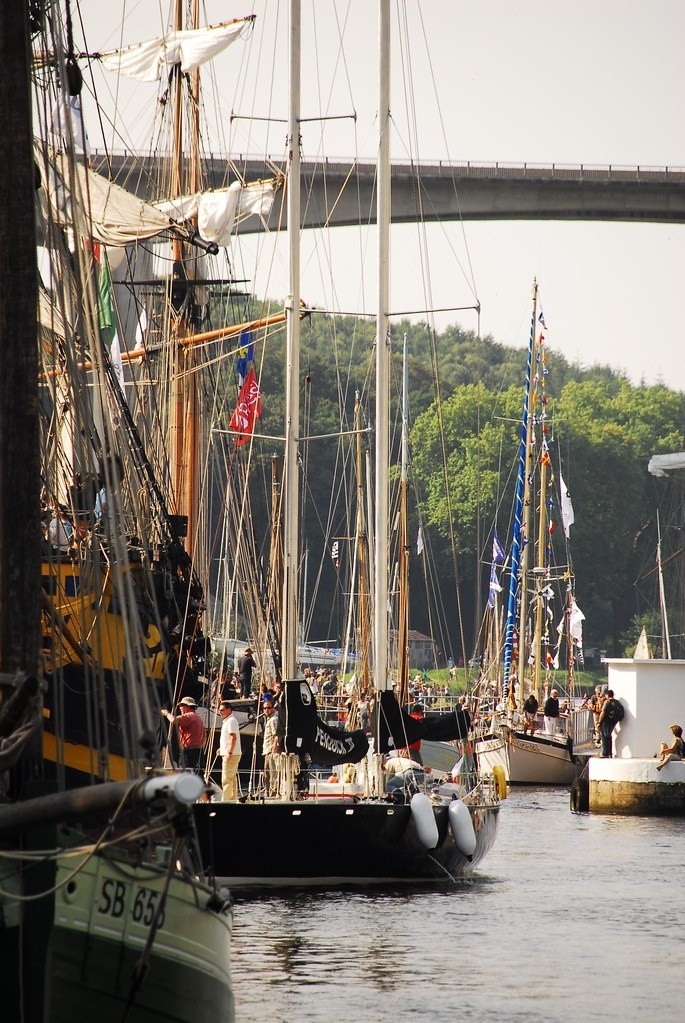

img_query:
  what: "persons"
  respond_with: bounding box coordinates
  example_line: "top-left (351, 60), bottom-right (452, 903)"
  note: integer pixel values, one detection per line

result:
top-left (522, 694), bottom-right (538, 737)
top-left (542, 689), bottom-right (562, 744)
top-left (160, 695), bottom-right (211, 804)
top-left (259, 700), bottom-right (282, 797)
top-left (400, 705), bottom-right (424, 766)
top-left (380, 756), bottom-right (425, 802)
top-left (207, 650), bottom-right (525, 728)
top-left (236, 647), bottom-right (257, 699)
top-left (39, 470), bottom-right (109, 557)
top-left (328, 765), bottom-right (357, 785)
top-left (596, 690), bottom-right (621, 759)
top-left (652, 723), bottom-right (684, 773)
top-left (563, 684), bottom-right (609, 741)
top-left (217, 702), bottom-right (245, 803)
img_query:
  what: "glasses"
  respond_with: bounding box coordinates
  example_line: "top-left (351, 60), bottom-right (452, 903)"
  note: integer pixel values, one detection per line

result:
top-left (263, 707), bottom-right (272, 710)
top-left (218, 709), bottom-right (227, 712)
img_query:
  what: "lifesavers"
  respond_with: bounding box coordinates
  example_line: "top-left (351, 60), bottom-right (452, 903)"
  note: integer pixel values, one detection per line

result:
top-left (492, 765), bottom-right (508, 800)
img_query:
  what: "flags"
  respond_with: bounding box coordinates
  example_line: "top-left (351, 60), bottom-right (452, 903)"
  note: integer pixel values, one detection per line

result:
top-left (228, 317), bottom-right (256, 391)
top-left (84, 238), bottom-right (128, 431)
top-left (228, 367), bottom-right (262, 446)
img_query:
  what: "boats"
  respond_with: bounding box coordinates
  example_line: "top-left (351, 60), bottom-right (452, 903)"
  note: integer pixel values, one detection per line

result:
top-left (162, 1), bottom-right (507, 895)
top-left (463, 270), bottom-right (594, 788)
top-left (0, 0), bottom-right (260, 1023)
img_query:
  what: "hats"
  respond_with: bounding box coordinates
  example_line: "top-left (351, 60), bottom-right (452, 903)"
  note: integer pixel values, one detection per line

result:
top-left (177, 697), bottom-right (198, 707)
